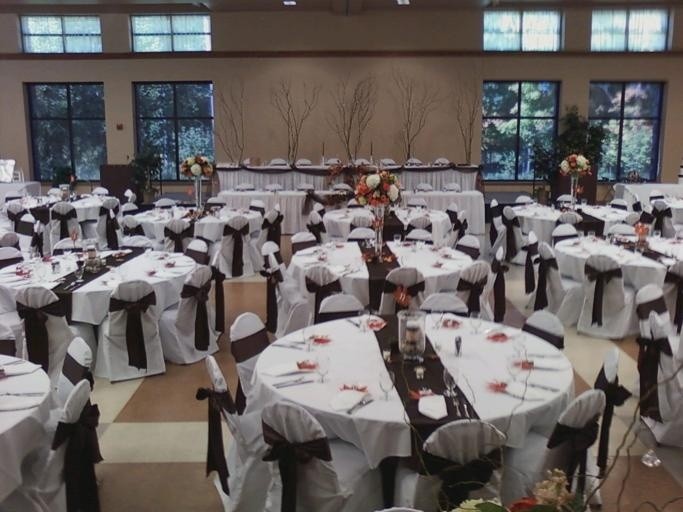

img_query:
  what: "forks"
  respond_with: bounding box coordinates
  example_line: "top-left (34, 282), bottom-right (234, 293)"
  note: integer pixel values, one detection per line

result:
top-left (451, 398), bottom-right (462, 418)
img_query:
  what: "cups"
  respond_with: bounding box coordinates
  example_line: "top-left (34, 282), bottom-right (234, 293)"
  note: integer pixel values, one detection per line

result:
top-left (396, 309), bottom-right (426, 361)
top-left (653, 230), bottom-right (660, 240)
top-left (587, 231), bottom-right (595, 239)
top-left (393, 234), bottom-right (401, 244)
top-left (576, 230), bottom-right (584, 240)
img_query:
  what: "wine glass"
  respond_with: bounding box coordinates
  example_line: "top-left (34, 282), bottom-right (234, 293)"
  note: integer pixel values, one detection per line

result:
top-left (429, 307), bottom-right (443, 331)
top-left (315, 355), bottom-right (332, 384)
top-left (302, 327), bottom-right (316, 352)
top-left (506, 357), bottom-right (523, 384)
top-left (377, 370), bottom-right (395, 403)
top-left (73, 266), bottom-right (85, 283)
top-left (468, 311), bottom-right (482, 335)
top-left (357, 309), bottom-right (370, 334)
top-left (442, 366), bottom-right (459, 399)
